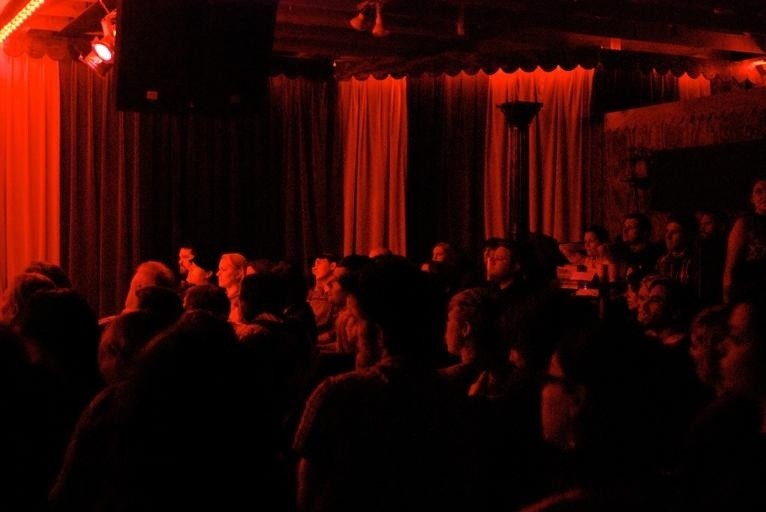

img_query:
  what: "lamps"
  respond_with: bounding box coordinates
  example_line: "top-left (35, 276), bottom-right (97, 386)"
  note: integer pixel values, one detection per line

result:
top-left (349, 1), bottom-right (392, 40)
top-left (74, 1), bottom-right (119, 81)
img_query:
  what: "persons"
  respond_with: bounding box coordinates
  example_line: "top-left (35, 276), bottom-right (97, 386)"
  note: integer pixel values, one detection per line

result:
top-left (2, 205), bottom-right (765, 512)
top-left (721, 172), bottom-right (766, 307)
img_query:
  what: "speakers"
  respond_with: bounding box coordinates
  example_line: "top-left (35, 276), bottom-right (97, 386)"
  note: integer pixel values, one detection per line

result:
top-left (116, 0), bottom-right (277, 113)
